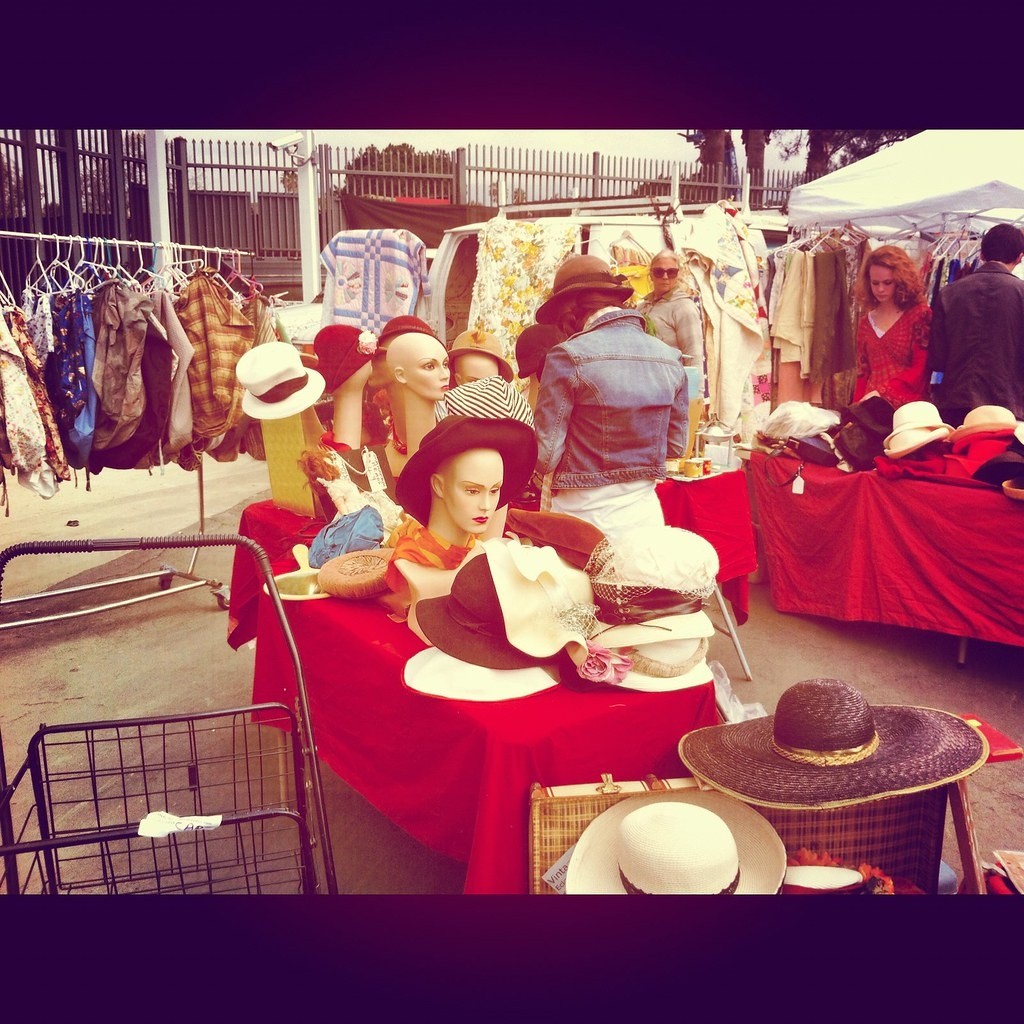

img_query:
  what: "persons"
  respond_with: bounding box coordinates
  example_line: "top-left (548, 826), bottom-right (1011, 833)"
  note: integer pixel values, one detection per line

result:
top-left (854, 246), bottom-right (931, 410)
top-left (640, 249), bottom-right (704, 400)
top-left (393, 415), bottom-right (536, 646)
top-left (529, 254), bottom-right (688, 533)
top-left (930, 224), bottom-right (1024, 432)
top-left (314, 317), bottom-right (515, 477)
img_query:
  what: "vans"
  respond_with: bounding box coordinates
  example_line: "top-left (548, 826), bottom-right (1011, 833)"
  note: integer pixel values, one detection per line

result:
top-left (418, 193), bottom-right (920, 351)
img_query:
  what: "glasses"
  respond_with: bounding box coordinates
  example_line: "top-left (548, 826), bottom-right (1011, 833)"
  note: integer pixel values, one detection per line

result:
top-left (651, 267), bottom-right (679, 279)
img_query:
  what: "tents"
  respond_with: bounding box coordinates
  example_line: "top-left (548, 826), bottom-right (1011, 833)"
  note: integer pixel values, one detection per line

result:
top-left (789, 130), bottom-right (1024, 239)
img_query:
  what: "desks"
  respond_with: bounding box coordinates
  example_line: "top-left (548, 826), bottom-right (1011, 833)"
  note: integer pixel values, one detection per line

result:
top-left (224, 438), bottom-right (759, 664)
top-left (249, 558), bottom-right (734, 894)
top-left (734, 431), bottom-right (1024, 674)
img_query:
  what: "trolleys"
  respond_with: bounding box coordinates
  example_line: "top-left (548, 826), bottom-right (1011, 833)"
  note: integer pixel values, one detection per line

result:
top-left (0, 533), bottom-right (340, 896)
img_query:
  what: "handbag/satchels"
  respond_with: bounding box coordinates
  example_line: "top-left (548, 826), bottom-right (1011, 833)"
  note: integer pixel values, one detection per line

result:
top-left (309, 506), bottom-right (385, 569)
top-left (764, 436), bottom-right (840, 487)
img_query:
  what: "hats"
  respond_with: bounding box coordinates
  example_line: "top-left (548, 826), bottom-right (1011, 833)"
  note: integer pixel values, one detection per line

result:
top-left (393, 415), bottom-right (538, 527)
top-left (679, 678), bottom-right (991, 806)
top-left (374, 316), bottom-right (447, 367)
top-left (839, 398), bottom-right (1024, 478)
top-left (399, 510), bottom-right (720, 702)
top-left (314, 324), bottom-right (380, 394)
top-left (445, 330), bottom-right (514, 385)
top-left (515, 324), bottom-right (572, 379)
top-left (536, 255), bottom-right (635, 325)
top-left (565, 788), bottom-right (786, 895)
top-left (235, 342), bottom-right (326, 420)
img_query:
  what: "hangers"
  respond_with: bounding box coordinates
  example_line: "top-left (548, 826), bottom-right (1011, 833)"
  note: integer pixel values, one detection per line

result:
top-left (774, 217), bottom-right (988, 266)
top-left (0, 232), bottom-right (269, 313)
